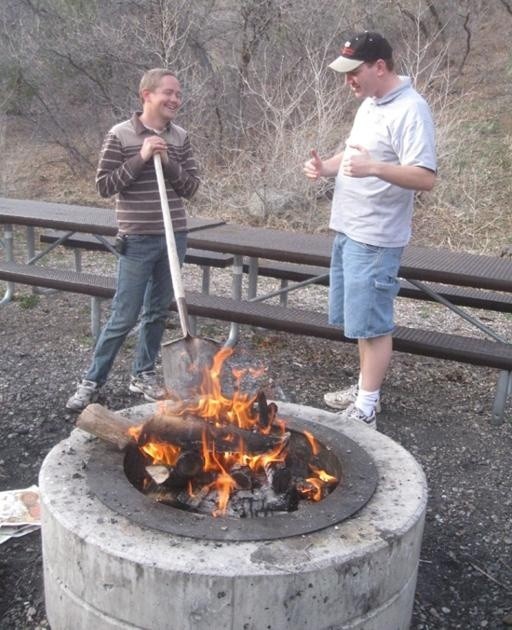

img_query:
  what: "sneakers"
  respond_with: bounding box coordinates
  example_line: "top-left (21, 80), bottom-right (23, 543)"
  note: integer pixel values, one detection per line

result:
top-left (128, 371), bottom-right (168, 403)
top-left (334, 405), bottom-right (379, 431)
top-left (64, 377), bottom-right (100, 412)
top-left (323, 384), bottom-right (383, 414)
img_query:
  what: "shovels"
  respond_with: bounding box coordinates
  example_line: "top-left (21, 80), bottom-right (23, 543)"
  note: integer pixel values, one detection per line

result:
top-left (153, 153), bottom-right (224, 401)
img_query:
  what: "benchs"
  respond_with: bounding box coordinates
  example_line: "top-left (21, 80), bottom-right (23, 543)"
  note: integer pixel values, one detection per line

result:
top-left (171, 292), bottom-right (512, 426)
top-left (0, 260), bottom-right (192, 341)
top-left (244, 254), bottom-right (512, 335)
top-left (40, 229), bottom-right (233, 295)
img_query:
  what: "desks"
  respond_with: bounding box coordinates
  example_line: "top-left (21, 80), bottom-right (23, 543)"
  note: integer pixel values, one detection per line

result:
top-left (186, 222), bottom-right (512, 347)
top-left (0, 197), bottom-right (225, 334)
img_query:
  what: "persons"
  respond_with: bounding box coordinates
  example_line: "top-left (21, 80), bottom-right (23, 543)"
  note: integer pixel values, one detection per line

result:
top-left (64, 65), bottom-right (204, 413)
top-left (302, 29), bottom-right (442, 430)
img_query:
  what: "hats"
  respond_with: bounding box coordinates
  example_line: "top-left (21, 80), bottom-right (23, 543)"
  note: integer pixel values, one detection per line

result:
top-left (327, 31), bottom-right (394, 73)
